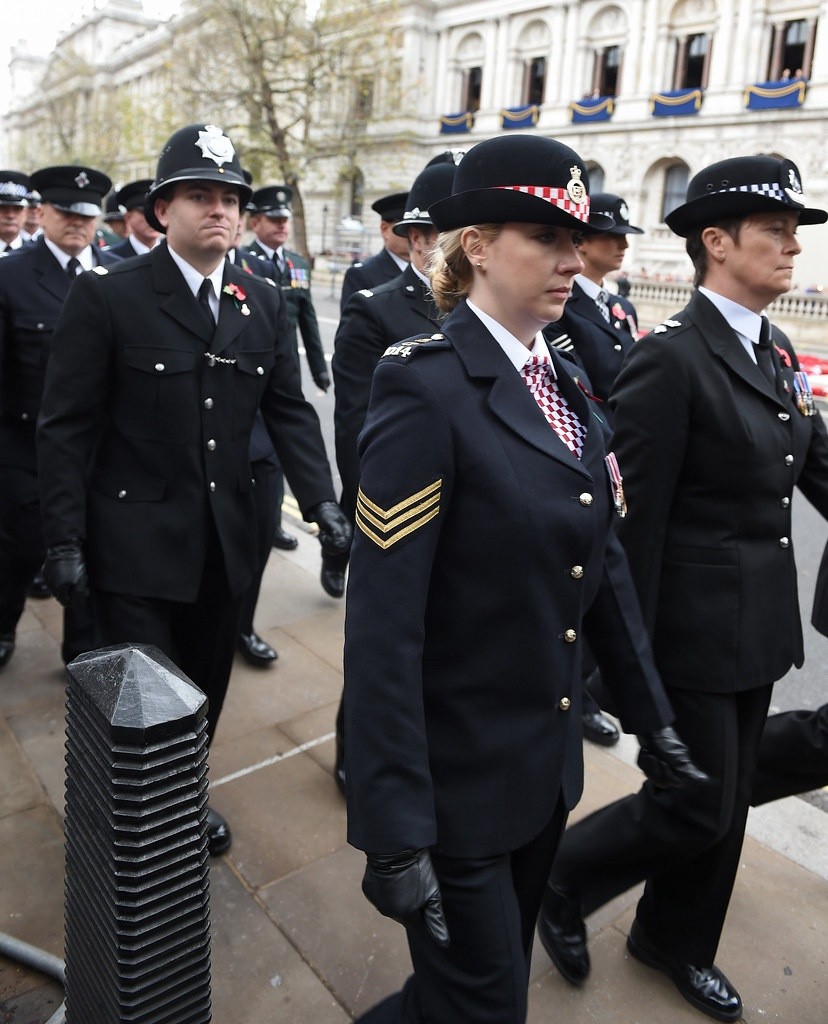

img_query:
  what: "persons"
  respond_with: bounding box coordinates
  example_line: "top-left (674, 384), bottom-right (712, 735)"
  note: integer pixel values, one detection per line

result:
top-left (319, 188), bottom-right (420, 597)
top-left (1, 163), bottom-right (127, 674)
top-left (747, 528), bottom-right (828, 810)
top-left (533, 149), bottom-right (828, 1024)
top-left (329, 160), bottom-right (506, 804)
top-left (540, 192), bottom-right (646, 746)
top-left (35, 120), bottom-right (355, 860)
top-left (229, 184), bottom-right (330, 549)
top-left (330, 134), bottom-right (711, 1023)
top-left (1, 169), bottom-right (165, 273)
top-left (204, 168), bottom-right (290, 662)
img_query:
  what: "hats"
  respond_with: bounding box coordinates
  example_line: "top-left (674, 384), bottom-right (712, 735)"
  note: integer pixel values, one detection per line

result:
top-left (24, 173), bottom-right (43, 208)
top-left (664, 152), bottom-right (828, 239)
top-left (29, 165), bottom-right (113, 218)
top-left (0, 170), bottom-right (30, 205)
top-left (117, 177), bottom-right (155, 210)
top-left (582, 194), bottom-right (644, 234)
top-left (371, 191), bottom-right (411, 222)
top-left (429, 133), bottom-right (618, 234)
top-left (245, 184), bottom-right (292, 217)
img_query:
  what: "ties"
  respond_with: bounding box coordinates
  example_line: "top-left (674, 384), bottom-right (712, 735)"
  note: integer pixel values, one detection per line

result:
top-left (271, 254), bottom-right (282, 274)
top-left (68, 258), bottom-right (79, 275)
top-left (199, 280), bottom-right (216, 331)
top-left (596, 291), bottom-right (612, 324)
top-left (519, 354), bottom-right (587, 460)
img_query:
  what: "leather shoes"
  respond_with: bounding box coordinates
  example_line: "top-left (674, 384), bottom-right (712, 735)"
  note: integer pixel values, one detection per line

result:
top-left (26, 576), bottom-right (49, 598)
top-left (625, 924), bottom-right (746, 1024)
top-left (540, 907), bottom-right (592, 985)
top-left (240, 632), bottom-right (280, 662)
top-left (202, 803), bottom-right (232, 855)
top-left (580, 711), bottom-right (621, 744)
top-left (273, 522), bottom-right (299, 548)
top-left (321, 566), bottom-right (346, 596)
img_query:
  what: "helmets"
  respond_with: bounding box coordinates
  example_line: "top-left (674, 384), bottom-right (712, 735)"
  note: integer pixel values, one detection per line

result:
top-left (392, 163), bottom-right (476, 238)
top-left (143, 123), bottom-right (253, 233)
top-left (104, 189), bottom-right (125, 224)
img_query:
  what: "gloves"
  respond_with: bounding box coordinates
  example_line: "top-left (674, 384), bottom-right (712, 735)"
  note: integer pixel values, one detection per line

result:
top-left (313, 501), bottom-right (351, 554)
top-left (43, 541), bottom-right (90, 606)
top-left (635, 723), bottom-right (711, 790)
top-left (362, 846), bottom-right (451, 948)
